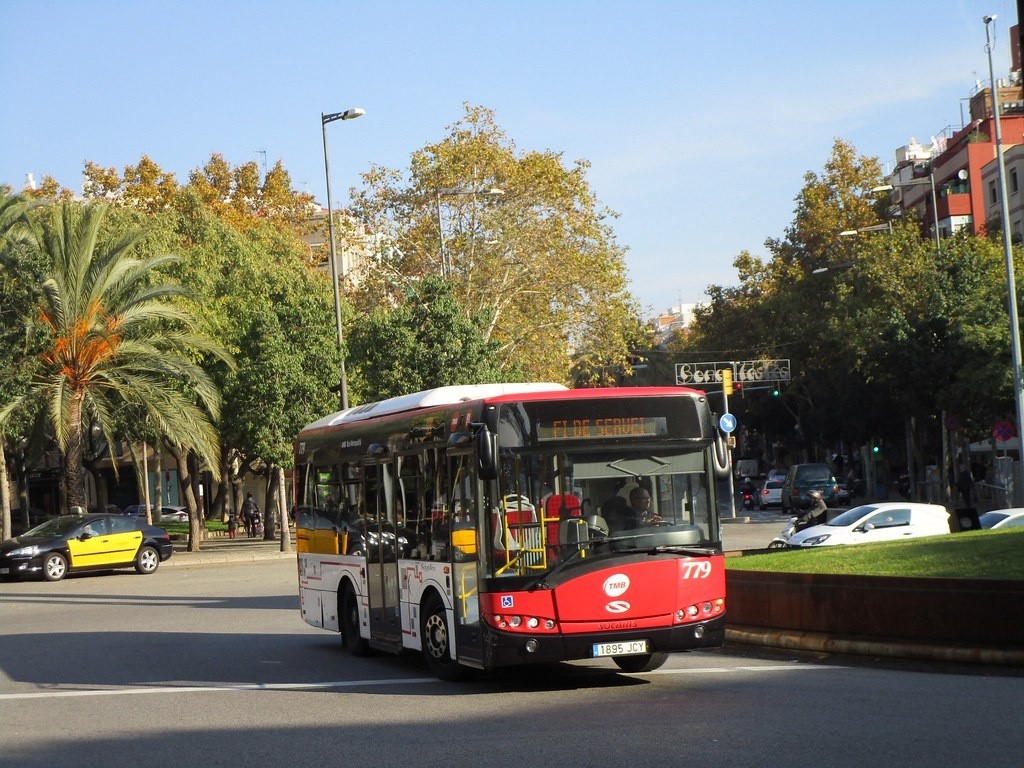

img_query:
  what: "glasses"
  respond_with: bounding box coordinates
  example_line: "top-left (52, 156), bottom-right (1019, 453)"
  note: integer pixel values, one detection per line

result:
top-left (637, 496), bottom-right (651, 500)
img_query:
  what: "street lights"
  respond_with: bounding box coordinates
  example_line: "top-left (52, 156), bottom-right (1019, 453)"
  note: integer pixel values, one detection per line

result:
top-left (321, 108), bottom-right (365, 409)
top-left (839, 220), bottom-right (916, 502)
top-left (436, 187), bottom-right (505, 280)
top-left (872, 173), bottom-right (959, 484)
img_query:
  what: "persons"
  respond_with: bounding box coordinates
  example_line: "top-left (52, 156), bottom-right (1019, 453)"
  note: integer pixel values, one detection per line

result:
top-left (738, 478), bottom-right (760, 511)
top-left (617, 486), bottom-right (664, 530)
top-left (291, 506), bottom-right (296, 527)
top-left (949, 463), bottom-right (991, 506)
top-left (240, 492), bottom-right (260, 537)
top-left (797, 489), bottom-right (829, 532)
top-left (227, 515), bottom-right (237, 539)
top-left (831, 452), bottom-right (863, 488)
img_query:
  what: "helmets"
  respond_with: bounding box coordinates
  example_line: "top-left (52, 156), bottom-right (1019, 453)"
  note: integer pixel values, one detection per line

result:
top-left (806, 489), bottom-right (820, 502)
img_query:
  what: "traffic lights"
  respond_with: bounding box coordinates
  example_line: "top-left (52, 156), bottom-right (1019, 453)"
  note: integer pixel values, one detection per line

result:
top-left (774, 381), bottom-right (780, 396)
top-left (737, 383), bottom-right (743, 398)
top-left (874, 446), bottom-right (879, 452)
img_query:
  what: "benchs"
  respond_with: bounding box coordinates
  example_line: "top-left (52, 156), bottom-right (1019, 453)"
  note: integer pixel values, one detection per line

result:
top-left (234, 514), bottom-right (282, 535)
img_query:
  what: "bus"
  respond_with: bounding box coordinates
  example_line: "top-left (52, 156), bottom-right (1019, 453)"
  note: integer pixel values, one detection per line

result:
top-left (292, 383), bottom-right (726, 681)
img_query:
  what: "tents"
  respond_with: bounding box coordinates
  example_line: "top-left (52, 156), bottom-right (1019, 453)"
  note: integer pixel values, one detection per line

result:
top-left (957, 434), bottom-right (1018, 458)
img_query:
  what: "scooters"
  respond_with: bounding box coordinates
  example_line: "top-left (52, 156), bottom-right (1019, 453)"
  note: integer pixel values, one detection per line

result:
top-left (767, 515), bottom-right (799, 548)
top-left (742, 490), bottom-right (756, 510)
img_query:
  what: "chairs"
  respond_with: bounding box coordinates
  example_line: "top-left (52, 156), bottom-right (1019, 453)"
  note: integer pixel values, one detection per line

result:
top-left (430, 491), bottom-right (629, 566)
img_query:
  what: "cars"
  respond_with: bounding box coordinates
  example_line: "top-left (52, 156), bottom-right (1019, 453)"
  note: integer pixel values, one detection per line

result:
top-left (784, 502), bottom-right (959, 549)
top-left (298, 506), bottom-right (409, 562)
top-left (0, 513), bottom-right (173, 581)
top-left (979, 508), bottom-right (1024, 530)
top-left (122, 505), bottom-right (190, 521)
top-left (735, 459), bottom-right (758, 480)
top-left (836, 477), bottom-right (851, 505)
top-left (756, 469), bottom-right (787, 509)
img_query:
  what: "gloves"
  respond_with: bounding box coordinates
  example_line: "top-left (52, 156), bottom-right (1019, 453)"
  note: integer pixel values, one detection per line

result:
top-left (792, 519), bottom-right (801, 528)
top-left (791, 515), bottom-right (797, 518)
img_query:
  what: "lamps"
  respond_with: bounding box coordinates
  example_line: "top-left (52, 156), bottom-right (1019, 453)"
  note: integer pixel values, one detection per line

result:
top-left (947, 509), bottom-right (983, 533)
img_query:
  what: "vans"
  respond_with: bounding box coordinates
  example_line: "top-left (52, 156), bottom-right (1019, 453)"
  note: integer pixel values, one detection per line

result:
top-left (781, 463), bottom-right (839, 514)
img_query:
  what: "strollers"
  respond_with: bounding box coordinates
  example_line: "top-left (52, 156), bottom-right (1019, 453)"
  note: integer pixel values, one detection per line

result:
top-left (253, 513), bottom-right (264, 537)
